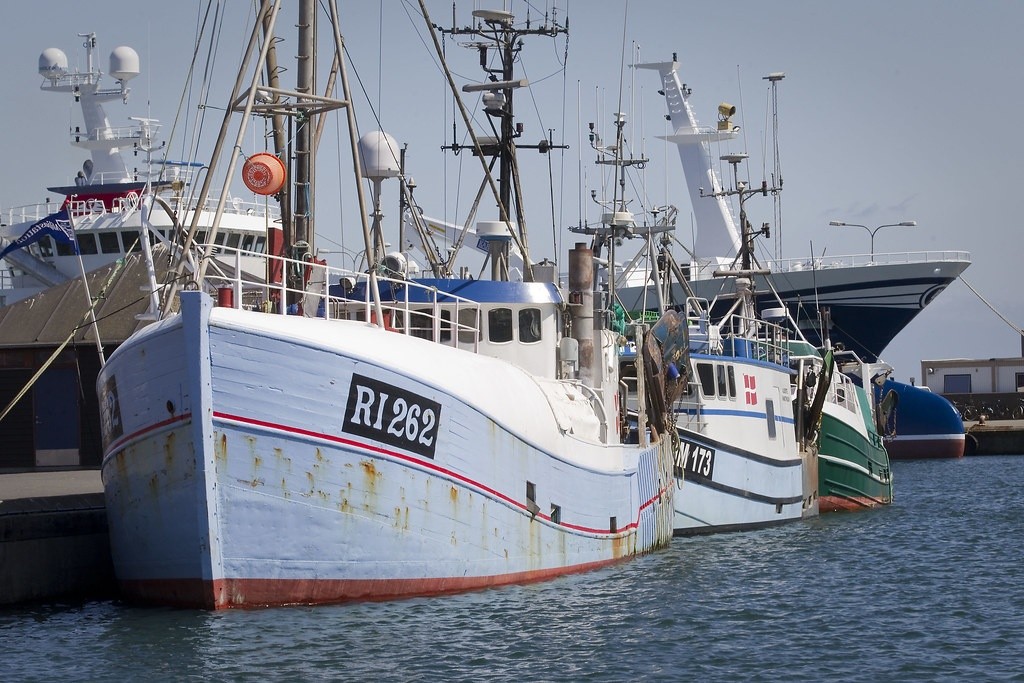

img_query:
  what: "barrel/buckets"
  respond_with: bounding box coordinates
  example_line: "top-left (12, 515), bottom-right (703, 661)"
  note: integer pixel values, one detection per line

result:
top-left (217, 287), bottom-right (234, 308)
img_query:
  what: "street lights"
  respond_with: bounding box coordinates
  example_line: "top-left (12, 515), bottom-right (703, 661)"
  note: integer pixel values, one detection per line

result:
top-left (828, 221), bottom-right (917, 264)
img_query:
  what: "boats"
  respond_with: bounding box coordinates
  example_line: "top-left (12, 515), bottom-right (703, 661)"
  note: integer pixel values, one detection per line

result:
top-left (700, 150), bottom-right (896, 509)
top-left (0, 0), bottom-right (974, 524)
top-left (568, 112), bottom-right (820, 533)
top-left (92, 0), bottom-right (679, 607)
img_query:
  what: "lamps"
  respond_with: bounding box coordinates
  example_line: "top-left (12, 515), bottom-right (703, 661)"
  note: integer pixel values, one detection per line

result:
top-left (339, 277), bottom-right (356, 294)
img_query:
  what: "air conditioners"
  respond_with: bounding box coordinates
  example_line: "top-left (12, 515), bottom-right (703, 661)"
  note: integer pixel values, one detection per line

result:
top-left (380, 252), bottom-right (407, 289)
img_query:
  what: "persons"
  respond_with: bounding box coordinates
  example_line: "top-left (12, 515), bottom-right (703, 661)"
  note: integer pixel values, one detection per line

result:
top-left (623, 422), bottom-right (634, 444)
top-left (805, 367), bottom-right (816, 403)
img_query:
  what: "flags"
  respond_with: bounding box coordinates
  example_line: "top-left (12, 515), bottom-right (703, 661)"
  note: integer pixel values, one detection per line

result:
top-left (0, 207), bottom-right (79, 260)
